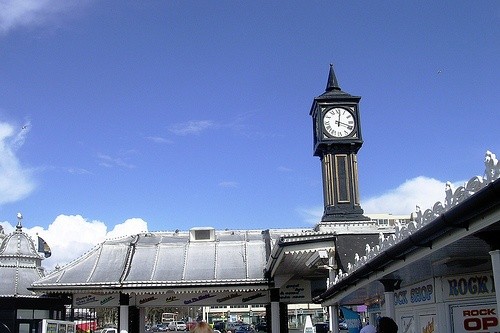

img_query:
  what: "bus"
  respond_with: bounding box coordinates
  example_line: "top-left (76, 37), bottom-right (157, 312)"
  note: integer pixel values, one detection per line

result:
top-left (65, 308), bottom-right (98, 333)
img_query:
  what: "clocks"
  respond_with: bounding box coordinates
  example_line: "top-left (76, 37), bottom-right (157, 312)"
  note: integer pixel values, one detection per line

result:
top-left (323, 107), bottom-right (355, 137)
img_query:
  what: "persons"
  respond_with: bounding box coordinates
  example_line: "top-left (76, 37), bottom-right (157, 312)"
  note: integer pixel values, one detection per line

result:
top-left (360, 325), bottom-right (377, 333)
top-left (189, 322), bottom-right (214, 333)
top-left (376, 316), bottom-right (398, 333)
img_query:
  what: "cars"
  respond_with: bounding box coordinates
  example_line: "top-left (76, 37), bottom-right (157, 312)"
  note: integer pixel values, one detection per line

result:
top-left (255, 322), bottom-right (266, 332)
top-left (339, 319), bottom-right (348, 330)
top-left (213, 320), bottom-right (256, 333)
top-left (100, 328), bottom-right (117, 333)
top-left (145, 312), bottom-right (187, 332)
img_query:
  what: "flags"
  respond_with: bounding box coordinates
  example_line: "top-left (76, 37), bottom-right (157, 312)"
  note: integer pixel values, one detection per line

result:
top-left (38, 237), bottom-right (51, 258)
